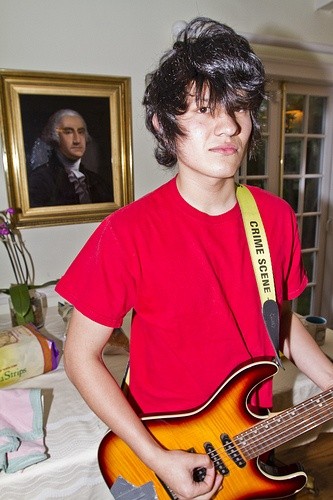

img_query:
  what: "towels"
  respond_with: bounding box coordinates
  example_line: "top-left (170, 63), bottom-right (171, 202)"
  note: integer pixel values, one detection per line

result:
top-left (0, 388), bottom-right (49, 473)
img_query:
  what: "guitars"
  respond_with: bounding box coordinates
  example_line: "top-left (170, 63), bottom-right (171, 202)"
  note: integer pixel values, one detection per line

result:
top-left (97, 362), bottom-right (333, 500)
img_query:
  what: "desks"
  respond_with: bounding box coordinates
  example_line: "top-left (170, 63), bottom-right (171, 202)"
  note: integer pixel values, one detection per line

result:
top-left (0, 305), bottom-right (333, 500)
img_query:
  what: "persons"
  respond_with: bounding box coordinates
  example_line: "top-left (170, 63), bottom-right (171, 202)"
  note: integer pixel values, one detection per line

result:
top-left (28, 110), bottom-right (114, 209)
top-left (55, 16), bottom-right (333, 500)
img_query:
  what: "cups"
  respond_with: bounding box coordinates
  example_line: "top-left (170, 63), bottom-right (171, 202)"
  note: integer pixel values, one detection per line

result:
top-left (303, 316), bottom-right (327, 346)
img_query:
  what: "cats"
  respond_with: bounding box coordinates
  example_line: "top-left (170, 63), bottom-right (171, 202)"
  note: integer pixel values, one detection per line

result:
top-left (58, 300), bottom-right (130, 355)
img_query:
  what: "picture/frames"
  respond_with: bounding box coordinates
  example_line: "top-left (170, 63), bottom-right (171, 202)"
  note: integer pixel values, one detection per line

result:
top-left (0, 69), bottom-right (135, 230)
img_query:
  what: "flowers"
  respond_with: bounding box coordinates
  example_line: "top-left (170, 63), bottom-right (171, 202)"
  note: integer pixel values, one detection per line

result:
top-left (0, 207), bottom-right (35, 317)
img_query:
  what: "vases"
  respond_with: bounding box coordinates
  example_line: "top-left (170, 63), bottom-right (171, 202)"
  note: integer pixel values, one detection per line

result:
top-left (8, 292), bottom-right (45, 330)
